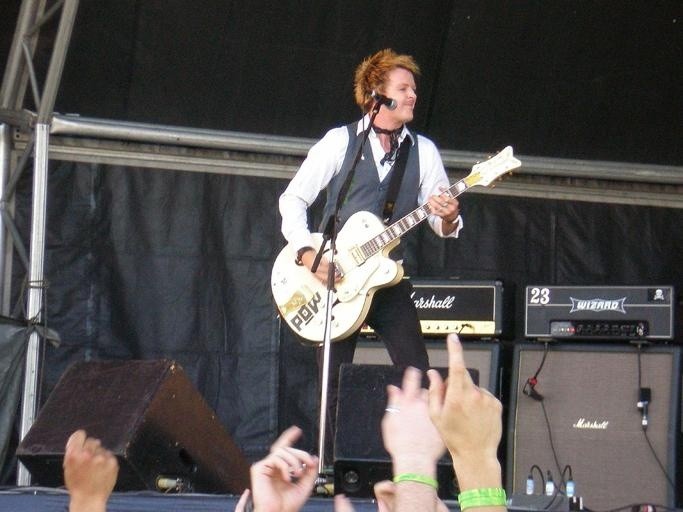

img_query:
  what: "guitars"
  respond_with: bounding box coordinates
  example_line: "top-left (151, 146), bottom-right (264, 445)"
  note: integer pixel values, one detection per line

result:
top-left (269, 146), bottom-right (522, 344)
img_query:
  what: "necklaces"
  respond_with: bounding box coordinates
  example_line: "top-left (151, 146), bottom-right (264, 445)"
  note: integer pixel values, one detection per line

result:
top-left (372, 124), bottom-right (404, 164)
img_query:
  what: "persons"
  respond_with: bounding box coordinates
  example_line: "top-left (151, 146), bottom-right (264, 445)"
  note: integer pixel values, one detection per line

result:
top-left (278, 49), bottom-right (466, 475)
top-left (62, 429), bottom-right (119, 512)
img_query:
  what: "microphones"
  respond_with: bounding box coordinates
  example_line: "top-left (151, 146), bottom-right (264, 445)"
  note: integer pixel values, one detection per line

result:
top-left (364, 89), bottom-right (398, 111)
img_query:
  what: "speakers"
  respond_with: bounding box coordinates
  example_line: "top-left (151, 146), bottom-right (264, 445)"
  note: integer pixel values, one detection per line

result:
top-left (15, 358), bottom-right (253, 491)
top-left (332, 361), bottom-right (480, 501)
top-left (506, 342), bottom-right (683, 511)
top-left (351, 340), bottom-right (504, 398)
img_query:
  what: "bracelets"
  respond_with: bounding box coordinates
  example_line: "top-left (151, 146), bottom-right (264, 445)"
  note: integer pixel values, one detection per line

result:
top-left (447, 215), bottom-right (461, 226)
top-left (295, 246), bottom-right (314, 266)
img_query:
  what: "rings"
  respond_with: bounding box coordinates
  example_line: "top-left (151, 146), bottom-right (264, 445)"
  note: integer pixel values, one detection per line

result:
top-left (438, 207), bottom-right (444, 211)
top-left (442, 202), bottom-right (448, 207)
